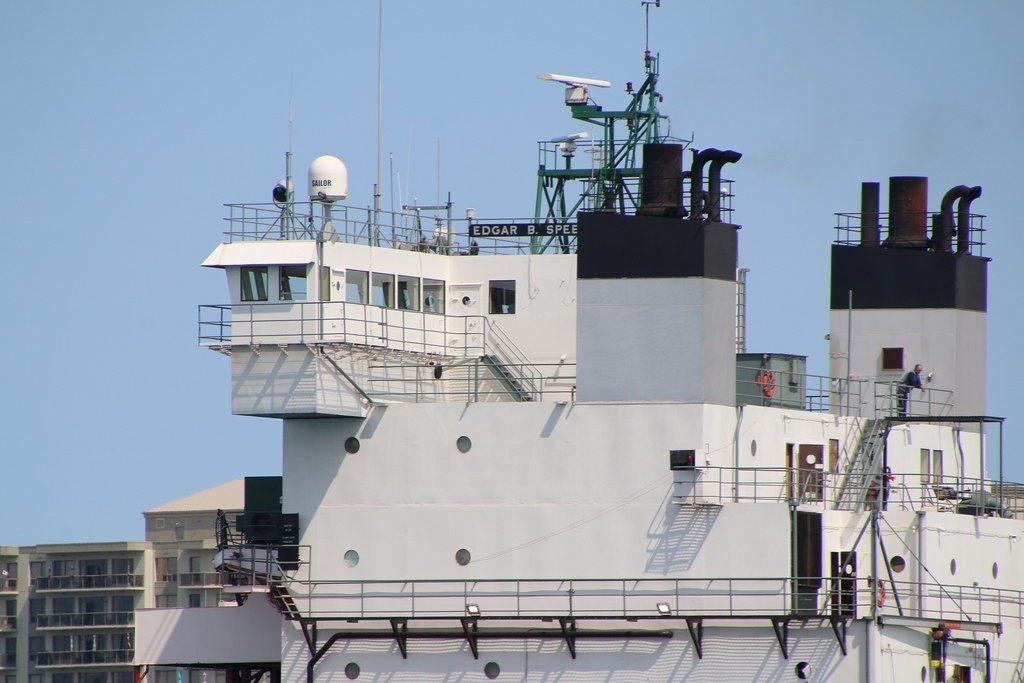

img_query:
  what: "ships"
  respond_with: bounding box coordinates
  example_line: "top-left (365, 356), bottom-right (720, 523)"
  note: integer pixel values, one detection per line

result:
top-left (132, 0), bottom-right (1024, 683)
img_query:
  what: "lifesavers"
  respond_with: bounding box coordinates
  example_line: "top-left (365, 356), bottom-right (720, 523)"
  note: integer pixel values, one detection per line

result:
top-left (762, 371), bottom-right (775, 397)
top-left (873, 579), bottom-right (887, 607)
